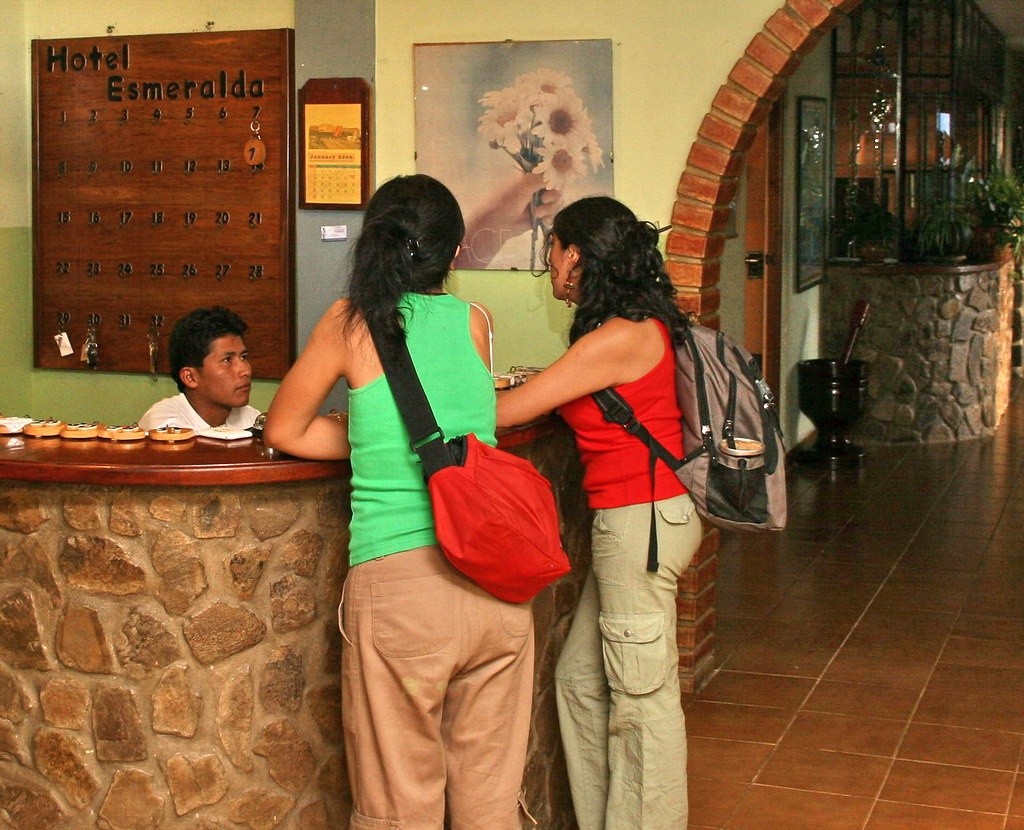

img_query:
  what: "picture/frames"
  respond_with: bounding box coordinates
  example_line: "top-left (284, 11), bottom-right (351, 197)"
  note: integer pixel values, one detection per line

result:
top-left (796, 95), bottom-right (831, 293)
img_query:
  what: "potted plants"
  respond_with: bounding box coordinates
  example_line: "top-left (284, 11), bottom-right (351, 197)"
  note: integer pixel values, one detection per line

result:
top-left (850, 169), bottom-right (1024, 266)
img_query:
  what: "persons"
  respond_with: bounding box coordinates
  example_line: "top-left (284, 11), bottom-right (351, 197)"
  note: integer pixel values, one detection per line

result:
top-left (262, 175), bottom-right (537, 830)
top-left (948, 140), bottom-right (970, 170)
top-left (453, 172), bottom-right (564, 272)
top-left (496, 195), bottom-right (706, 830)
top-left (136, 304), bottom-right (264, 441)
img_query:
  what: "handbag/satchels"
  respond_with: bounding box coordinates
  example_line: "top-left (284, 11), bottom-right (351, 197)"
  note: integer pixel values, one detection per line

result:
top-left (424, 432), bottom-right (571, 604)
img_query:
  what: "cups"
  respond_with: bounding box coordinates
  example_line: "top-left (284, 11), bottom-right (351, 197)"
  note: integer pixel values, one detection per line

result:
top-left (717, 436), bottom-right (766, 457)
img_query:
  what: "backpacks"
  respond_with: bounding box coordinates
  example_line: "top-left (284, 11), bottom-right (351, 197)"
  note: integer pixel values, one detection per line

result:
top-left (591, 301), bottom-right (787, 533)
